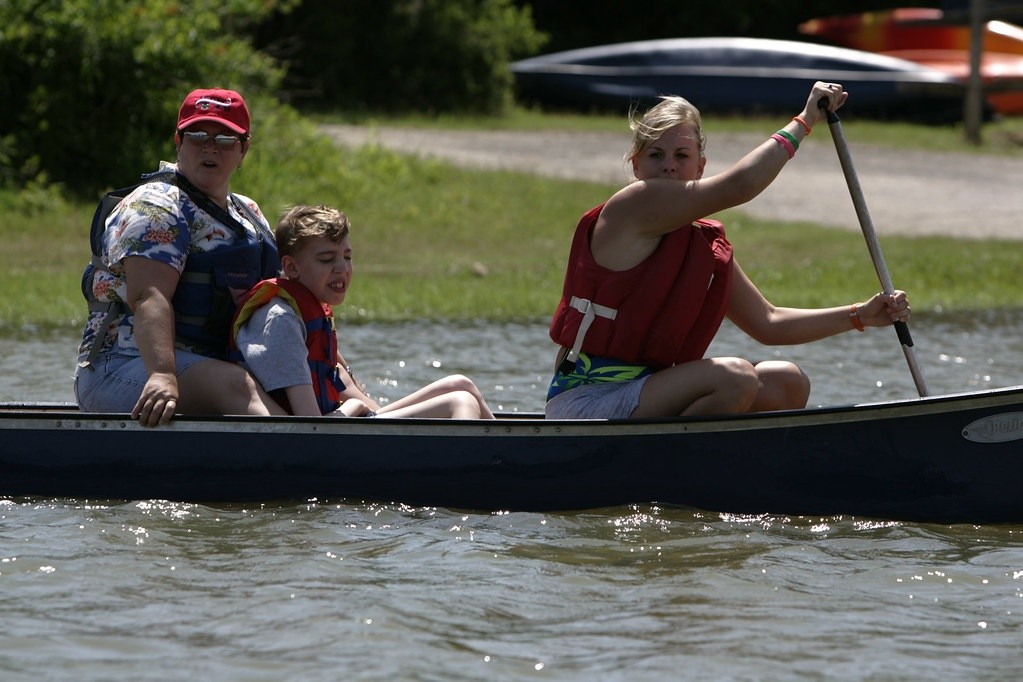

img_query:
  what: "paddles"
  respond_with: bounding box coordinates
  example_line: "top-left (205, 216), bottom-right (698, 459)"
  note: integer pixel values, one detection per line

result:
top-left (816, 92), bottom-right (930, 399)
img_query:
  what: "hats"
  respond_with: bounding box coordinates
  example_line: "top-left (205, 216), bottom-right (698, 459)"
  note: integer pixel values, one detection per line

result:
top-left (177, 86), bottom-right (251, 135)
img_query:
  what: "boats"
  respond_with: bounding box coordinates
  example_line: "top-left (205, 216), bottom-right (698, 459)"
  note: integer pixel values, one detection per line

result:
top-left (501, 8), bottom-right (1023, 127)
top-left (0, 383), bottom-right (1023, 524)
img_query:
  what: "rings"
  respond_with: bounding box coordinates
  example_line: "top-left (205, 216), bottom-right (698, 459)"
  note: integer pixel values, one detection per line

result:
top-left (828, 85), bottom-right (832, 89)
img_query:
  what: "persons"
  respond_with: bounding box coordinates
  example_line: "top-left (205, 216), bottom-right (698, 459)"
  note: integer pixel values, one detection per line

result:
top-left (232, 203), bottom-right (496, 420)
top-left (546, 80), bottom-right (912, 421)
top-left (72, 84), bottom-right (290, 429)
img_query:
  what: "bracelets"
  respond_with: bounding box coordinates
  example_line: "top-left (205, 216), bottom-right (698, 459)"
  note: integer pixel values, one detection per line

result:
top-left (770, 129), bottom-right (799, 159)
top-left (851, 302), bottom-right (865, 333)
top-left (792, 116), bottom-right (811, 136)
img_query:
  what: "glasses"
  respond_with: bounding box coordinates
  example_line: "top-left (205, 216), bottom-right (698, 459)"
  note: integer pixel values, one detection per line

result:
top-left (183, 130), bottom-right (242, 149)
